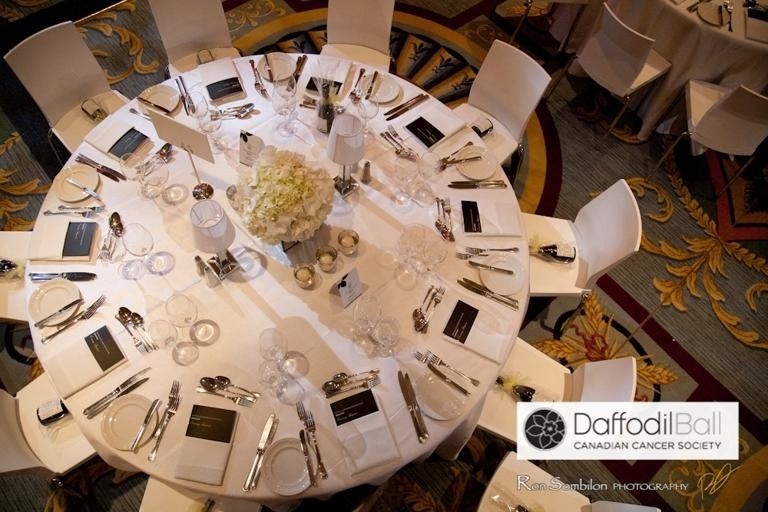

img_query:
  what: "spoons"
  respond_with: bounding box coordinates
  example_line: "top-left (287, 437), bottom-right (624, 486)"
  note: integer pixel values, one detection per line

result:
top-left (414, 287), bottom-right (439, 332)
top-left (434, 197), bottom-right (442, 231)
top-left (336, 105), bottom-right (345, 114)
top-left (215, 375), bottom-right (262, 398)
top-left (100, 211), bottom-right (121, 260)
top-left (412, 284), bottom-right (434, 322)
top-left (332, 368), bottom-right (381, 382)
top-left (106, 222), bottom-right (123, 257)
top-left (380, 133), bottom-right (401, 154)
top-left (199, 376), bottom-right (257, 402)
top-left (118, 306), bottom-right (153, 354)
top-left (160, 142), bottom-right (172, 155)
top-left (321, 375), bottom-right (378, 392)
top-left (131, 311), bottom-right (160, 351)
top-left (438, 199), bottom-right (448, 241)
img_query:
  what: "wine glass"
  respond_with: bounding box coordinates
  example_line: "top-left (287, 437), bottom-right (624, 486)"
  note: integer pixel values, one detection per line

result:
top-left (147, 319), bottom-right (199, 364)
top-left (353, 294), bottom-right (403, 361)
top-left (122, 221), bottom-right (174, 274)
top-left (390, 154), bottom-right (420, 205)
top-left (271, 85), bottom-right (296, 137)
top-left (198, 104), bottom-right (223, 155)
top-left (119, 152), bottom-right (161, 200)
top-left (276, 71), bottom-right (297, 104)
top-left (417, 152), bottom-right (440, 205)
top-left (97, 234), bottom-right (145, 280)
top-left (139, 153), bottom-right (184, 203)
top-left (399, 222), bottom-right (449, 288)
top-left (165, 293), bottom-right (217, 344)
top-left (118, 260), bottom-right (165, 319)
top-left (256, 327), bottom-right (298, 372)
top-left (184, 91), bottom-right (208, 127)
top-left (257, 360), bottom-right (289, 397)
top-left (358, 93), bottom-right (379, 145)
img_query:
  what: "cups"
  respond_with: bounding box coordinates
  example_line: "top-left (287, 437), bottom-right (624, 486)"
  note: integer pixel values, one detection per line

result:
top-left (293, 262), bottom-right (316, 288)
top-left (316, 244), bottom-right (337, 272)
top-left (337, 228), bottom-right (359, 256)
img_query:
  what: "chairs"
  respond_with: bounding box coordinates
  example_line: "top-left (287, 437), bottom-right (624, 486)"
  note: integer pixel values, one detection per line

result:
top-left (3, 20), bottom-right (130, 166)
top-left (544, 1), bottom-right (672, 140)
top-left (451, 38), bottom-right (551, 187)
top-left (319, 1), bottom-right (395, 73)
top-left (0, 371), bottom-right (97, 475)
top-left (508, 0), bottom-right (588, 53)
top-left (147, 0), bottom-right (242, 78)
top-left (644, 76), bottom-right (767, 200)
top-left (136, 476), bottom-right (262, 512)
top-left (477, 450), bottom-right (659, 511)
top-left (0, 231), bottom-right (31, 323)
top-left (478, 336), bottom-right (637, 463)
top-left (521, 178), bottom-right (642, 339)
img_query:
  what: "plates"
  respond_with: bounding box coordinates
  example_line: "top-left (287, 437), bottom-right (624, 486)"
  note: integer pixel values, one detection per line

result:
top-left (52, 164), bottom-right (99, 202)
top-left (361, 72), bottom-right (401, 105)
top-left (455, 145), bottom-right (497, 180)
top-left (260, 437), bottom-right (319, 497)
top-left (256, 51), bottom-right (297, 82)
top-left (100, 393), bottom-right (159, 452)
top-left (480, 252), bottom-right (526, 296)
top-left (27, 278), bottom-right (81, 327)
top-left (414, 373), bottom-right (469, 421)
top-left (696, 0), bottom-right (730, 26)
top-left (137, 84), bottom-right (180, 116)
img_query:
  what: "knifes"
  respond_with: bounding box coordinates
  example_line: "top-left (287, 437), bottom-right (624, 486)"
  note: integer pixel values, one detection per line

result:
top-left (263, 54), bottom-right (273, 81)
top-left (179, 76), bottom-right (195, 113)
top-left (717, 5), bottom-right (724, 26)
top-left (299, 429), bottom-right (319, 488)
top-left (136, 96), bottom-right (170, 114)
top-left (451, 180), bottom-right (505, 184)
top-left (364, 70), bottom-right (379, 100)
top-left (463, 277), bottom-right (518, 304)
top-left (82, 366), bottom-right (149, 415)
top-left (405, 373), bottom-right (429, 439)
top-left (287, 54), bottom-right (308, 91)
top-left (468, 261), bottom-right (513, 276)
top-left (76, 158), bottom-right (120, 182)
top-left (447, 184), bottom-right (506, 190)
top-left (384, 94), bottom-right (423, 115)
top-left (66, 177), bottom-right (101, 202)
top-left (74, 152), bottom-right (127, 180)
top-left (175, 78), bottom-right (189, 116)
top-left (446, 156), bottom-right (482, 165)
top-left (242, 412), bottom-right (274, 492)
top-left (398, 370), bottom-right (425, 444)
top-left (427, 363), bottom-right (472, 398)
top-left (456, 280), bottom-right (519, 311)
top-left (27, 271), bottom-right (96, 283)
top-left (127, 398), bottom-right (161, 452)
top-left (251, 419), bottom-right (279, 490)
top-left (88, 377), bottom-right (149, 419)
top-left (386, 95), bottom-right (429, 121)
top-left (34, 299), bottom-right (84, 328)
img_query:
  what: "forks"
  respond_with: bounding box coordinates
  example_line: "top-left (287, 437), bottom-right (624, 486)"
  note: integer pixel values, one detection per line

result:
top-left (250, 60), bottom-right (261, 93)
top-left (40, 293), bottom-right (107, 345)
top-left (301, 94), bottom-right (316, 102)
top-left (154, 379), bottom-right (181, 438)
top-left (114, 313), bottom-right (147, 356)
top-left (148, 397), bottom-right (180, 462)
top-left (466, 247), bottom-right (519, 253)
top-left (444, 198), bottom-right (452, 242)
top-left (255, 68), bottom-right (269, 98)
top-left (347, 67), bottom-right (366, 104)
top-left (325, 377), bottom-right (381, 398)
top-left (415, 351), bottom-right (429, 363)
top-left (42, 202), bottom-right (106, 219)
top-left (428, 288), bottom-right (447, 323)
top-left (425, 349), bottom-right (480, 386)
top-left (723, 0), bottom-right (735, 32)
top-left (386, 124), bottom-right (404, 141)
top-left (296, 400), bottom-right (329, 480)
top-left (455, 252), bottom-right (479, 259)
top-left (195, 385), bottom-right (254, 407)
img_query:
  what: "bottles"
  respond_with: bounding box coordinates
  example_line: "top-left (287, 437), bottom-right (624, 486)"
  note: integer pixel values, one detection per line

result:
top-left (196, 49), bottom-right (215, 65)
top-left (201, 498), bottom-right (215, 512)
top-left (35, 397), bottom-right (70, 427)
top-left (0, 260), bottom-right (18, 278)
top-left (529, 243), bottom-right (577, 264)
top-left (318, 83), bottom-right (335, 133)
top-left (80, 97), bottom-right (109, 125)
top-left (471, 118), bottom-right (494, 138)
top-left (497, 376), bottom-right (555, 402)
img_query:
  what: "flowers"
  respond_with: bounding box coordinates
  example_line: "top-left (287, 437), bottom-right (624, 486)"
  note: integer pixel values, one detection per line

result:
top-left (236, 145), bottom-right (336, 245)
